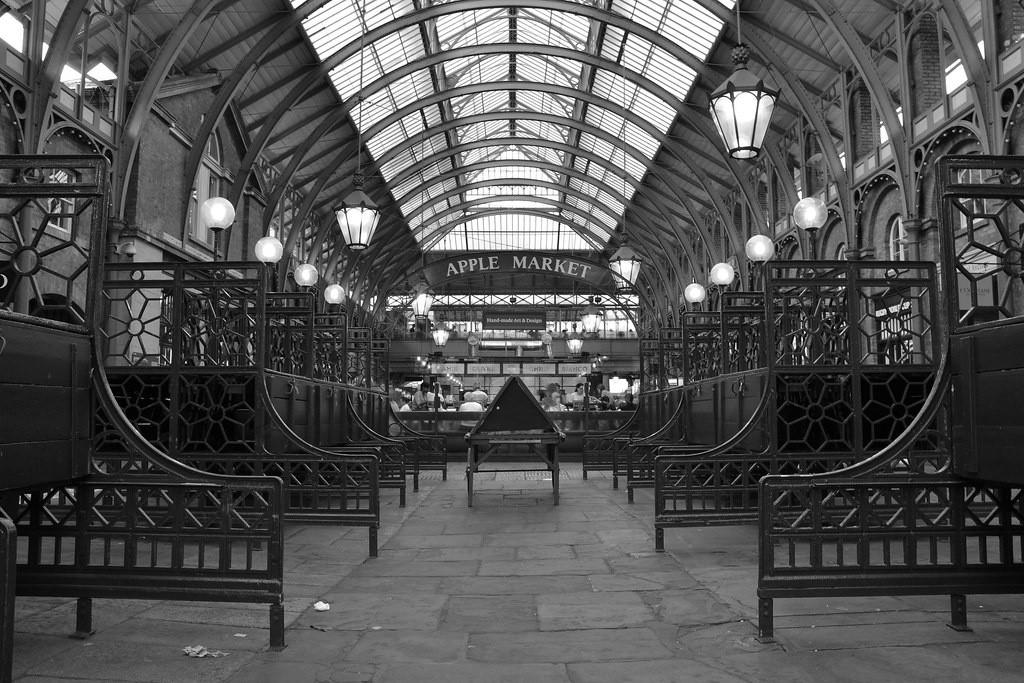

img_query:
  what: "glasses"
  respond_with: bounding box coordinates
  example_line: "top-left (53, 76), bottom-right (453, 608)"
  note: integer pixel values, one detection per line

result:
top-left (426, 387), bottom-right (428, 389)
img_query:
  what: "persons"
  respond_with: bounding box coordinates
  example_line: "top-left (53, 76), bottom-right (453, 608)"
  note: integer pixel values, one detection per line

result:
top-left (451, 326), bottom-right (456, 333)
top-left (617, 394), bottom-right (637, 410)
top-left (597, 384), bottom-right (613, 409)
top-left (544, 383), bottom-right (557, 410)
top-left (413, 382), bottom-right (429, 411)
top-left (410, 324), bottom-right (415, 337)
top-left (529, 329), bottom-right (635, 339)
top-left (555, 382), bottom-right (560, 391)
top-left (459, 381), bottom-right (488, 412)
top-left (570, 383), bottom-right (590, 403)
top-left (399, 397), bottom-right (412, 411)
top-left (545, 392), bottom-right (568, 412)
top-left (427, 382), bottom-right (446, 411)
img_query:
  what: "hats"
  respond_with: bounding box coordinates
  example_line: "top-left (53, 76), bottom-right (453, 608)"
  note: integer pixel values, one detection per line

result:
top-left (421, 382), bottom-right (429, 387)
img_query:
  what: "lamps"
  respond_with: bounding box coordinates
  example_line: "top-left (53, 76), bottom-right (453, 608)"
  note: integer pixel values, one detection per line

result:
top-left (607, 43), bottom-right (642, 292)
top-left (410, 115), bottom-right (437, 333)
top-left (431, 200), bottom-right (451, 348)
top-left (565, 251), bottom-right (584, 356)
top-left (294, 264), bottom-right (318, 292)
top-left (324, 284), bottom-right (345, 325)
top-left (86, 51), bottom-right (118, 83)
top-left (685, 283), bottom-right (706, 304)
top-left (711, 263), bottom-right (735, 295)
top-left (745, 235), bottom-right (774, 265)
top-left (578, 160), bottom-right (605, 335)
top-left (793, 198), bottom-right (830, 260)
top-left (199, 197), bottom-right (235, 261)
top-left (330, 0), bottom-right (383, 250)
top-left (255, 237), bottom-right (283, 291)
top-left (705, 0), bottom-right (782, 160)
